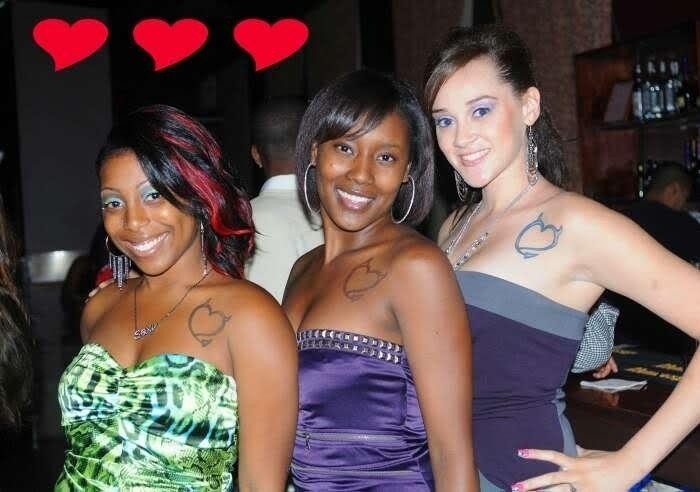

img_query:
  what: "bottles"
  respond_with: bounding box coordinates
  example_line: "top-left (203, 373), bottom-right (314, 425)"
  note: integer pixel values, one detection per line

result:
top-left (630, 53), bottom-right (696, 123)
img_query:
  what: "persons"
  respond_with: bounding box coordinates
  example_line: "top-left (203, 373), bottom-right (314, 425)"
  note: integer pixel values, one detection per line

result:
top-left (276, 67), bottom-right (484, 492)
top-left (243, 99), bottom-right (326, 306)
top-left (55, 103), bottom-right (299, 492)
top-left (1, 213), bottom-right (144, 492)
top-left (421, 21), bottom-right (700, 492)
top-left (538, 154), bottom-right (699, 453)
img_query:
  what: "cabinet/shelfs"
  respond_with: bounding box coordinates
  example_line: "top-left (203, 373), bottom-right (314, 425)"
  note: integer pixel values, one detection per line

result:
top-left (569, 1), bottom-right (700, 211)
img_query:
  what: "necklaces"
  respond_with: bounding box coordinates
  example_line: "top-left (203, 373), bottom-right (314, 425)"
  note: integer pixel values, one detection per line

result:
top-left (131, 269), bottom-right (216, 339)
